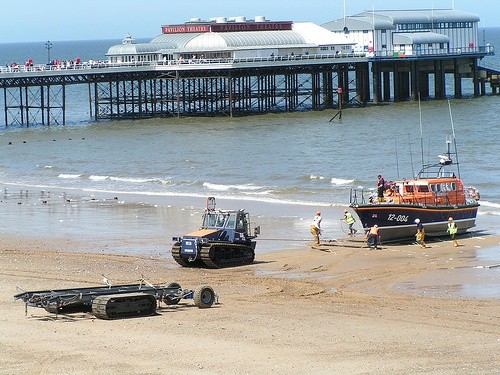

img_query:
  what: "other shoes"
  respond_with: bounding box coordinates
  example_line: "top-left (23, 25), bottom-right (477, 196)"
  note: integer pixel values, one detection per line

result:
top-left (421, 243), bottom-right (426, 248)
top-left (367, 248), bottom-right (371, 250)
top-left (375, 247), bottom-right (377, 251)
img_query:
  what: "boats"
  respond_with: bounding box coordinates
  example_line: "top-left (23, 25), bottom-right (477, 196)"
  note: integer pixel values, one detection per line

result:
top-left (351, 141), bottom-right (480, 245)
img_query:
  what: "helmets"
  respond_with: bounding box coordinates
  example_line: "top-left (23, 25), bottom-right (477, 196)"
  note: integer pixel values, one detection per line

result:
top-left (316, 211), bottom-right (321, 215)
top-left (374, 225), bottom-right (378, 228)
top-left (448, 217), bottom-right (453, 220)
top-left (415, 219), bottom-right (420, 224)
top-left (344, 210), bottom-right (348, 212)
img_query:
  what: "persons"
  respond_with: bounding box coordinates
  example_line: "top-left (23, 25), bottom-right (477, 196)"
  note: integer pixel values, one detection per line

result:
top-left (414, 218), bottom-right (426, 249)
top-left (0, 47), bottom-right (342, 73)
top-left (364, 224), bottom-right (383, 250)
top-left (310, 210), bottom-right (323, 246)
top-left (446, 216), bottom-right (459, 247)
top-left (374, 173), bottom-right (405, 205)
top-left (340, 209), bottom-right (358, 235)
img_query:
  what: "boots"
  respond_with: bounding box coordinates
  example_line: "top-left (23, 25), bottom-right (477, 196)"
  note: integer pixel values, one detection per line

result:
top-left (348, 230), bottom-right (353, 235)
top-left (353, 228), bottom-right (357, 234)
top-left (454, 240), bottom-right (458, 247)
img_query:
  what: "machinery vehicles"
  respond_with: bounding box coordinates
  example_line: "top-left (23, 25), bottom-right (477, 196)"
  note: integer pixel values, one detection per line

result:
top-left (173, 196), bottom-right (261, 269)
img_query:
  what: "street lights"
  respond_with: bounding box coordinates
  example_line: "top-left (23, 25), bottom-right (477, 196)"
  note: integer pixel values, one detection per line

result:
top-left (44, 40), bottom-right (54, 64)
